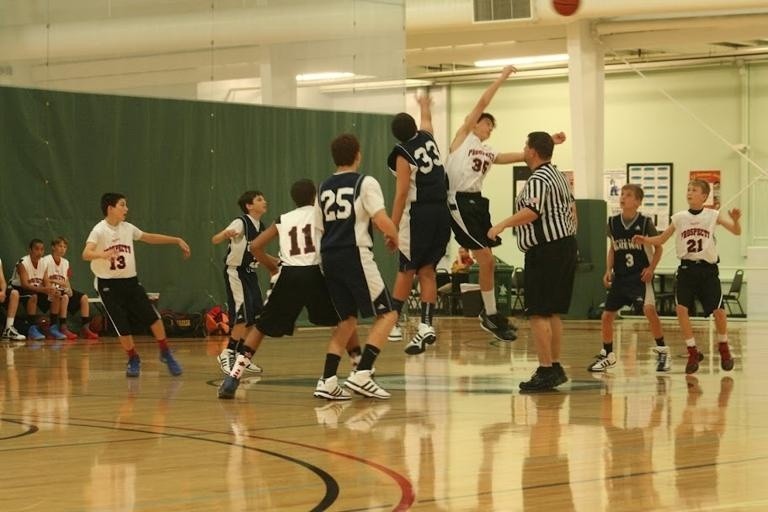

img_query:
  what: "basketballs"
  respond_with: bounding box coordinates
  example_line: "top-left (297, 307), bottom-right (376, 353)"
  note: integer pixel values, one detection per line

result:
top-left (553, 0), bottom-right (578, 15)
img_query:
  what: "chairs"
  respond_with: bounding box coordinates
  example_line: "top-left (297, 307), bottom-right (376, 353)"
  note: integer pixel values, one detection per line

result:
top-left (722, 267), bottom-right (749, 318)
top-left (509, 266), bottom-right (526, 317)
top-left (397, 262), bottom-right (468, 318)
top-left (651, 272), bottom-right (674, 315)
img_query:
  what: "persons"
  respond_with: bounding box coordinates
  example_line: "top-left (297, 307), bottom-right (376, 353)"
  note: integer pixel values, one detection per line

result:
top-left (631, 178), bottom-right (743, 374)
top-left (8, 239), bottom-right (68, 341)
top-left (486, 132), bottom-right (578, 390)
top-left (217, 177), bottom-right (376, 400)
top-left (312, 133), bottom-right (399, 401)
top-left (0, 259), bottom-right (26, 341)
top-left (42, 236), bottom-right (100, 340)
top-left (383, 93), bottom-right (451, 355)
top-left (586, 184), bottom-right (672, 372)
top-left (82, 192), bottom-right (191, 376)
top-left (211, 189), bottom-right (280, 376)
top-left (450, 246), bottom-right (474, 273)
top-left (443, 65), bottom-right (566, 343)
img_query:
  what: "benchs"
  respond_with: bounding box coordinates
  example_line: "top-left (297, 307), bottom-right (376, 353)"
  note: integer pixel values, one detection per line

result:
top-left (86, 291), bottom-right (163, 336)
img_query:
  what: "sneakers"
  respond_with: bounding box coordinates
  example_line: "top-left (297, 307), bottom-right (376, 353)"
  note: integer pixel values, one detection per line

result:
top-left (652, 345), bottom-right (672, 376)
top-left (2, 326), bottom-right (27, 340)
top-left (386, 326), bottom-right (404, 341)
top-left (587, 348), bottom-right (616, 372)
top-left (216, 349), bottom-right (264, 376)
top-left (126, 354), bottom-right (140, 377)
top-left (313, 375), bottom-right (352, 400)
top-left (344, 355), bottom-right (392, 400)
top-left (160, 348), bottom-right (183, 376)
top-left (719, 341), bottom-right (733, 370)
top-left (404, 323), bottom-right (436, 354)
top-left (80, 323), bottom-right (99, 339)
top-left (48, 325), bottom-right (78, 340)
top-left (28, 326), bottom-right (46, 340)
top-left (519, 367), bottom-right (568, 391)
top-left (685, 346), bottom-right (704, 374)
top-left (217, 375), bottom-right (240, 398)
top-left (479, 305), bottom-right (518, 342)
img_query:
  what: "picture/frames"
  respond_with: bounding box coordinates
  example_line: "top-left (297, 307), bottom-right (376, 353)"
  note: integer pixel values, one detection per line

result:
top-left (626, 162), bottom-right (675, 235)
